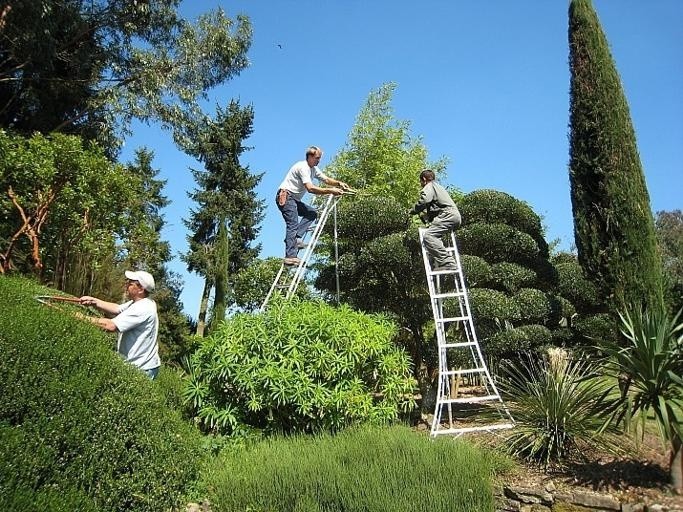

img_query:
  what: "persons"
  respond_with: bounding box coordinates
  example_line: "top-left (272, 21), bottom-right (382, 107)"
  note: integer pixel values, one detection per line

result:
top-left (73, 270), bottom-right (161, 382)
top-left (275, 146), bottom-right (350, 266)
top-left (408, 169), bottom-right (462, 271)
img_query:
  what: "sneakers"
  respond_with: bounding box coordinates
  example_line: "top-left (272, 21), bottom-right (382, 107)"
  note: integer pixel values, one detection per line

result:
top-left (285, 257), bottom-right (306, 265)
top-left (434, 263), bottom-right (456, 270)
top-left (297, 241), bottom-right (308, 248)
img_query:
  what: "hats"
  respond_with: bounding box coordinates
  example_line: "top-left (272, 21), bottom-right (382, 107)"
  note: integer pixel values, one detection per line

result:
top-left (125, 271), bottom-right (155, 293)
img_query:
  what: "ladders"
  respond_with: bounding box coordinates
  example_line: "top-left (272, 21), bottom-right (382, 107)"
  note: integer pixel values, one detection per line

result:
top-left (260, 185), bottom-right (339, 315)
top-left (417, 226), bottom-right (517, 442)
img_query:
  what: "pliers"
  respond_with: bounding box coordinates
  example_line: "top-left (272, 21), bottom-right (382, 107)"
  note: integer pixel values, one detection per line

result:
top-left (33, 296), bottom-right (97, 321)
